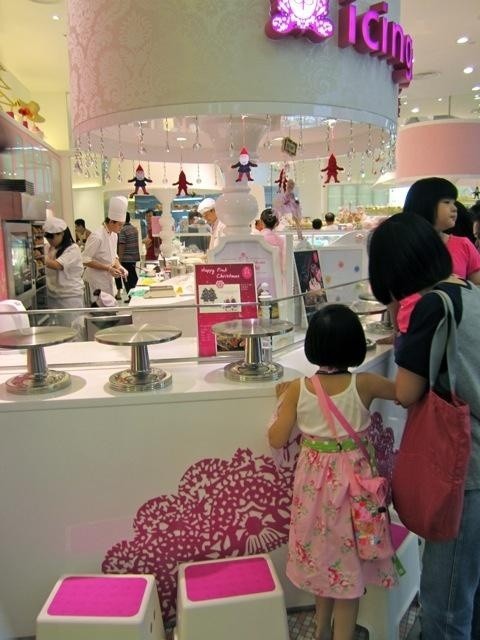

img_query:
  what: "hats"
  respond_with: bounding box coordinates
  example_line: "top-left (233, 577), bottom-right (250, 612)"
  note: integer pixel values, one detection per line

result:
top-left (198, 198), bottom-right (215, 214)
top-left (108, 196), bottom-right (128, 221)
top-left (43, 217), bottom-right (67, 233)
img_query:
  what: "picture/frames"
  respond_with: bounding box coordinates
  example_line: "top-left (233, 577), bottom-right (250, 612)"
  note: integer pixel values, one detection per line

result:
top-left (311, 244), bottom-right (368, 323)
top-left (206, 233), bottom-right (291, 327)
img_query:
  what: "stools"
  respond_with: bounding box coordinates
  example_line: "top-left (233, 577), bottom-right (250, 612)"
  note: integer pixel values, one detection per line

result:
top-left (175, 557), bottom-right (293, 639)
top-left (33, 572), bottom-right (166, 640)
top-left (313, 521), bottom-right (421, 640)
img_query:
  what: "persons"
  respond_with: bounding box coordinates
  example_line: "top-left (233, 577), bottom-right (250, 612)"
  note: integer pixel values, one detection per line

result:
top-left (396, 176), bottom-right (479, 331)
top-left (179, 198), bottom-right (226, 248)
top-left (115, 232), bottom-right (123, 300)
top-left (143, 209), bottom-right (161, 260)
top-left (118, 212), bottom-right (140, 303)
top-left (268, 305), bottom-right (396, 640)
top-left (82, 214), bottom-right (128, 330)
top-left (369, 212), bottom-right (480, 640)
top-left (42, 218), bottom-right (85, 343)
top-left (75, 219), bottom-right (91, 250)
top-left (255, 209), bottom-right (285, 262)
top-left (312, 219), bottom-right (323, 230)
top-left (322, 212), bottom-right (338, 231)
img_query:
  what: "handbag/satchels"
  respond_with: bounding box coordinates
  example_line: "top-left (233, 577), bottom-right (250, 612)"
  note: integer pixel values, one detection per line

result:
top-left (348, 478), bottom-right (395, 560)
top-left (391, 393), bottom-right (472, 544)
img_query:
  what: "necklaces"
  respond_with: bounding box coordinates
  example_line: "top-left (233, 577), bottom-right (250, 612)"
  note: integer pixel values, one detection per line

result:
top-left (315, 366), bottom-right (352, 375)
